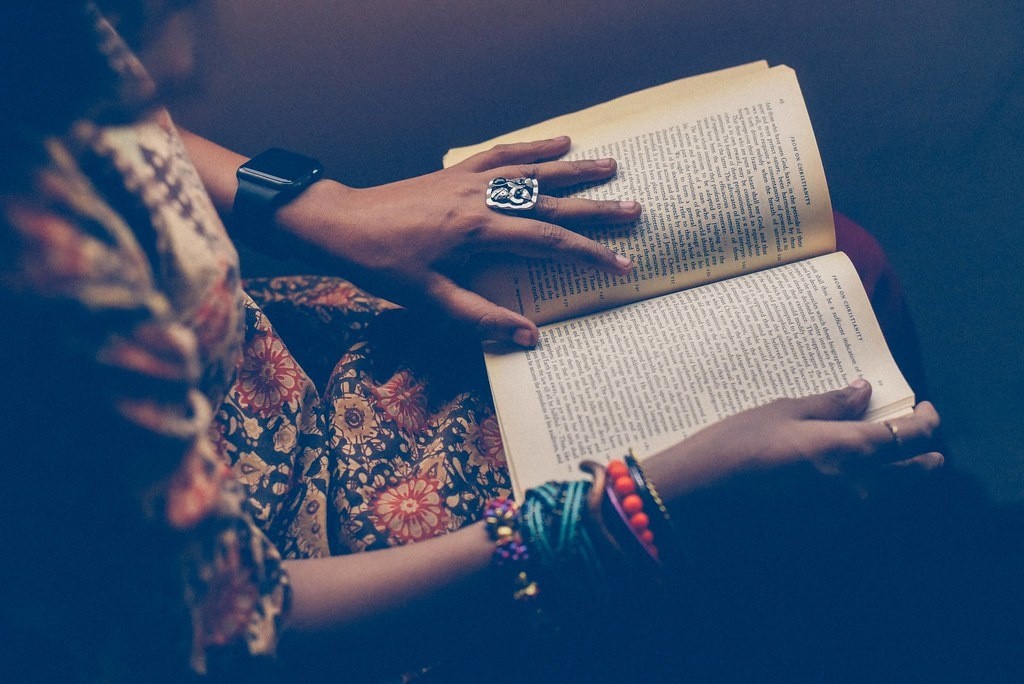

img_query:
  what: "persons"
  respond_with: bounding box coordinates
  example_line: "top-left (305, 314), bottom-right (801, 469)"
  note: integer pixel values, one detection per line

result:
top-left (0, 0), bottom-right (945, 684)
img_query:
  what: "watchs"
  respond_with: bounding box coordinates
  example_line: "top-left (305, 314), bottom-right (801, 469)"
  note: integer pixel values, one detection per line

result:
top-left (230, 148), bottom-right (322, 250)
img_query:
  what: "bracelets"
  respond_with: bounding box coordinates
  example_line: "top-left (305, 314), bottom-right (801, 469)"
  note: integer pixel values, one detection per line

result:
top-left (485, 452), bottom-right (677, 617)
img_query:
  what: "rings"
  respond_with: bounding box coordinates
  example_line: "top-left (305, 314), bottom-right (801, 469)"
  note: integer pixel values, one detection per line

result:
top-left (885, 421), bottom-right (905, 452)
top-left (487, 177), bottom-right (539, 211)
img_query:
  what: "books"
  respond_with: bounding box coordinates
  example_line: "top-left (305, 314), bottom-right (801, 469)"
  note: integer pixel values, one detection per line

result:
top-left (443, 61), bottom-right (919, 506)
